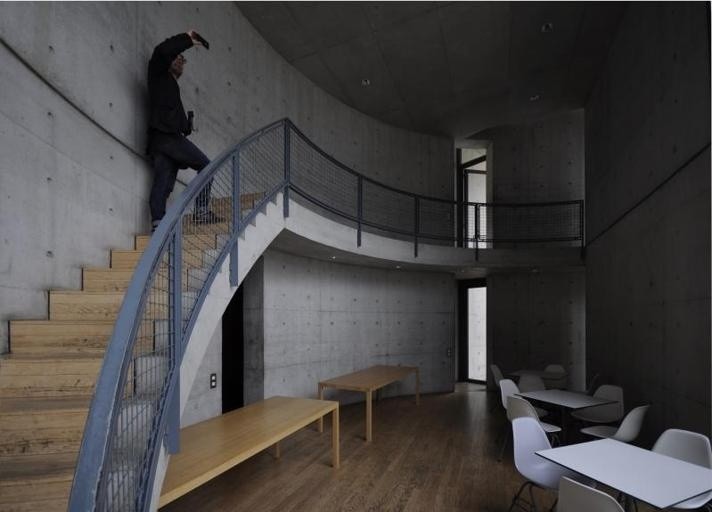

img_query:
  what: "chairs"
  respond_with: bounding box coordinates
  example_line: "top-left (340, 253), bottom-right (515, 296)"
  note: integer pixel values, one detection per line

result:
top-left (488, 361), bottom-right (711, 512)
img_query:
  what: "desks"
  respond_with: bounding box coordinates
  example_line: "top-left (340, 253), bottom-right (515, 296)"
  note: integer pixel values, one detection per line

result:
top-left (156, 365), bottom-right (421, 510)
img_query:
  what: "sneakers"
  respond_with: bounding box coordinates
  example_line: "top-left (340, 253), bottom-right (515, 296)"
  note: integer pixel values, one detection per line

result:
top-left (192, 210), bottom-right (227, 224)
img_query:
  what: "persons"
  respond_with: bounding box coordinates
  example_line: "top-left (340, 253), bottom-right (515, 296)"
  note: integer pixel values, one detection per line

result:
top-left (145, 30), bottom-right (226, 234)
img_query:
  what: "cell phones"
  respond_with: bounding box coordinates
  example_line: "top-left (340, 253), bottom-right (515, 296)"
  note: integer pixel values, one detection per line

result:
top-left (197, 35), bottom-right (208, 49)
